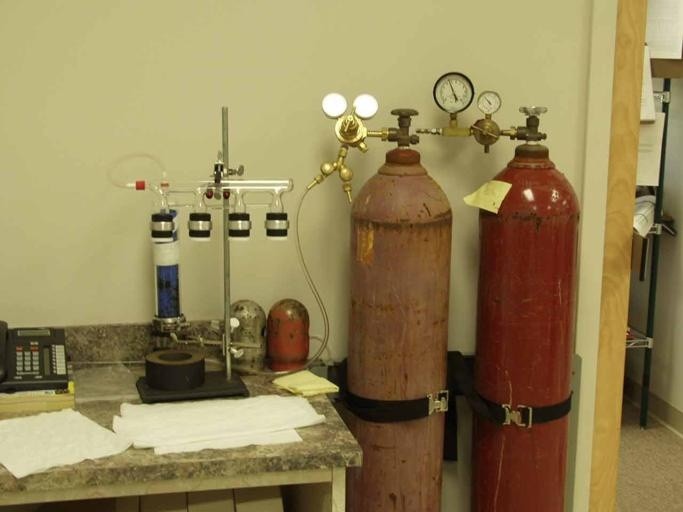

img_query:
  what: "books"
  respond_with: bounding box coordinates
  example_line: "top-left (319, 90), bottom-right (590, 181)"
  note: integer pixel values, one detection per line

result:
top-left (0, 364), bottom-right (74, 414)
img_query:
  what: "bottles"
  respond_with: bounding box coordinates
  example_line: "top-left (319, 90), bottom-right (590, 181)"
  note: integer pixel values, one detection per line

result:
top-left (154, 208), bottom-right (181, 317)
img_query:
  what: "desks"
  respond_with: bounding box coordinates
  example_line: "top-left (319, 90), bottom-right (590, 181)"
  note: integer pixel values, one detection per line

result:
top-left (0, 322), bottom-right (363, 512)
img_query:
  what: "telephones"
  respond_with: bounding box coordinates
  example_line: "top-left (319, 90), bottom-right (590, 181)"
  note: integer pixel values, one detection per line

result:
top-left (0, 321), bottom-right (67, 394)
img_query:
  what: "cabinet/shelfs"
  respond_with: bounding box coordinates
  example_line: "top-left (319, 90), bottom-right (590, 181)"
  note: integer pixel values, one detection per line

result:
top-left (626, 79), bottom-right (677, 427)
